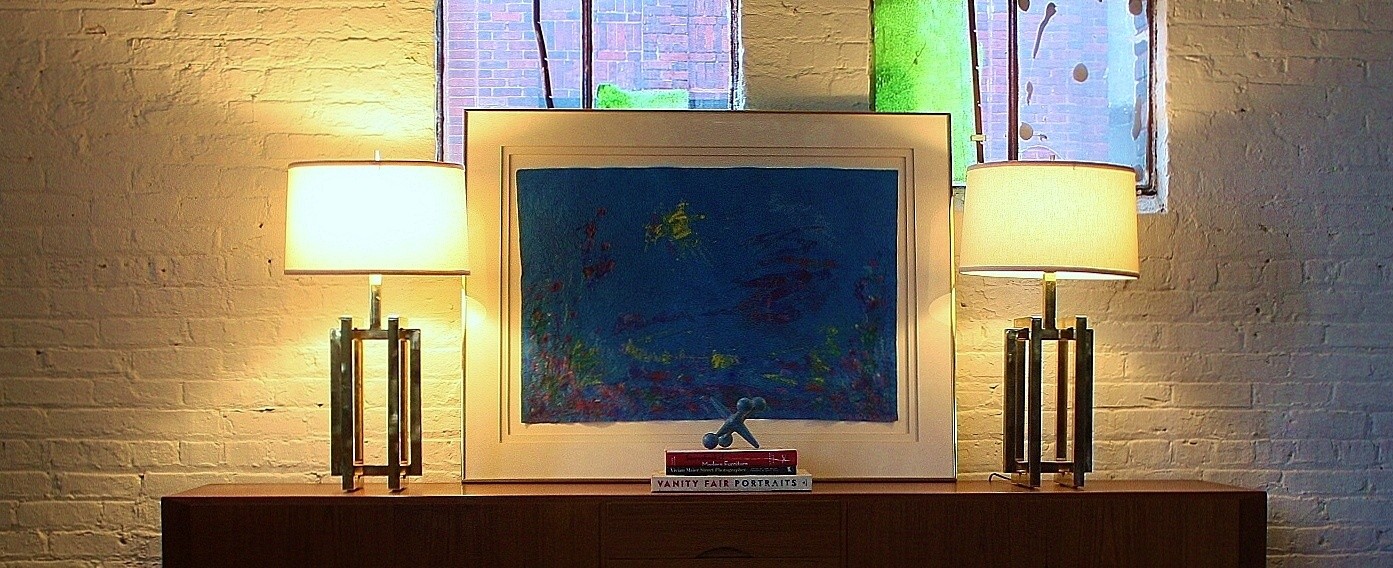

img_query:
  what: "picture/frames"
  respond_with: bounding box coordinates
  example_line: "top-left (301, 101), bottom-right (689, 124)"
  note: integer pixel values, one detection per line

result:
top-left (462, 108), bottom-right (959, 484)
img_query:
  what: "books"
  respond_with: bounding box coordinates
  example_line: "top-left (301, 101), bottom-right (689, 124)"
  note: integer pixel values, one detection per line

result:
top-left (651, 448), bottom-right (812, 492)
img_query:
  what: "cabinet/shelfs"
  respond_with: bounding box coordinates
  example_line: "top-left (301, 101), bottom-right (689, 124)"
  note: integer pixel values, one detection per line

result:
top-left (160, 478), bottom-right (1269, 568)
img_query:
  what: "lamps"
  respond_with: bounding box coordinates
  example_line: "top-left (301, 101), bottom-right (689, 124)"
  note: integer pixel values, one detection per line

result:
top-left (280, 148), bottom-right (470, 491)
top-left (958, 152), bottom-right (1141, 487)
